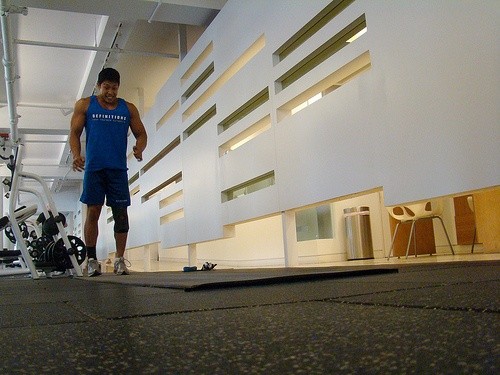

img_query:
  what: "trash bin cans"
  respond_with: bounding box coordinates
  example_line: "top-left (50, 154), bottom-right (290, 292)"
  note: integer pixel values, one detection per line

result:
top-left (343, 206), bottom-right (376, 260)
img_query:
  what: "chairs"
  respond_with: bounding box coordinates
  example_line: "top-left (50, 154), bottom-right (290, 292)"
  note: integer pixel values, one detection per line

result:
top-left (388, 202), bottom-right (454, 261)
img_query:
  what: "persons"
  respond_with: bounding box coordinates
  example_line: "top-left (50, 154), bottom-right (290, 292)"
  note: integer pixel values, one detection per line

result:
top-left (70, 68), bottom-right (147, 276)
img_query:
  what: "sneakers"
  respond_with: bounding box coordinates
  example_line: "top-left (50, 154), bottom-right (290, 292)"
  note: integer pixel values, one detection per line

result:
top-left (87, 260), bottom-right (102, 277)
top-left (114, 257), bottom-right (131, 275)
